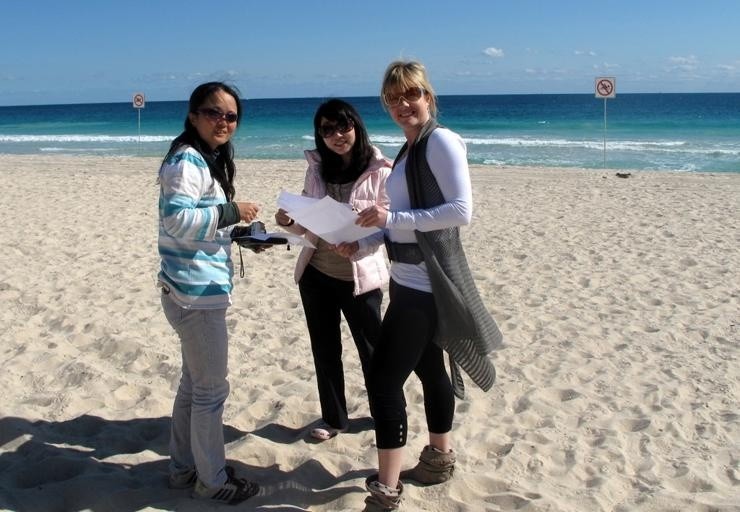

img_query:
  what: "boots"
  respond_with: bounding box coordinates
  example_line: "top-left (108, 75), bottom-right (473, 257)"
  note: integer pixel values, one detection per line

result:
top-left (361, 474), bottom-right (405, 512)
top-left (400, 445), bottom-right (455, 486)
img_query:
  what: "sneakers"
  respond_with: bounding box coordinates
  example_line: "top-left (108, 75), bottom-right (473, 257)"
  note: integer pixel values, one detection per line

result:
top-left (168, 465), bottom-right (235, 489)
top-left (192, 474), bottom-right (265, 506)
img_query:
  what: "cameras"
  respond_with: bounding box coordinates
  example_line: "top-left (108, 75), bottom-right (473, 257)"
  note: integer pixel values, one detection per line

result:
top-left (230, 221), bottom-right (265, 238)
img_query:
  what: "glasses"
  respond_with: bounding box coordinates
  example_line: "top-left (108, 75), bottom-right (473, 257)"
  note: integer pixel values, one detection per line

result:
top-left (382, 87), bottom-right (429, 106)
top-left (196, 108), bottom-right (238, 122)
top-left (317, 118), bottom-right (355, 139)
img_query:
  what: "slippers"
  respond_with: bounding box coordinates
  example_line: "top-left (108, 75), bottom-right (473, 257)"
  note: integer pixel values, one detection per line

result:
top-left (310, 421), bottom-right (350, 440)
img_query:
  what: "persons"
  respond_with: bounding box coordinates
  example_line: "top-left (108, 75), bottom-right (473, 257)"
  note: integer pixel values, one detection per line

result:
top-left (156, 82), bottom-right (260, 505)
top-left (328, 59), bottom-right (475, 511)
top-left (270, 98), bottom-right (392, 437)
